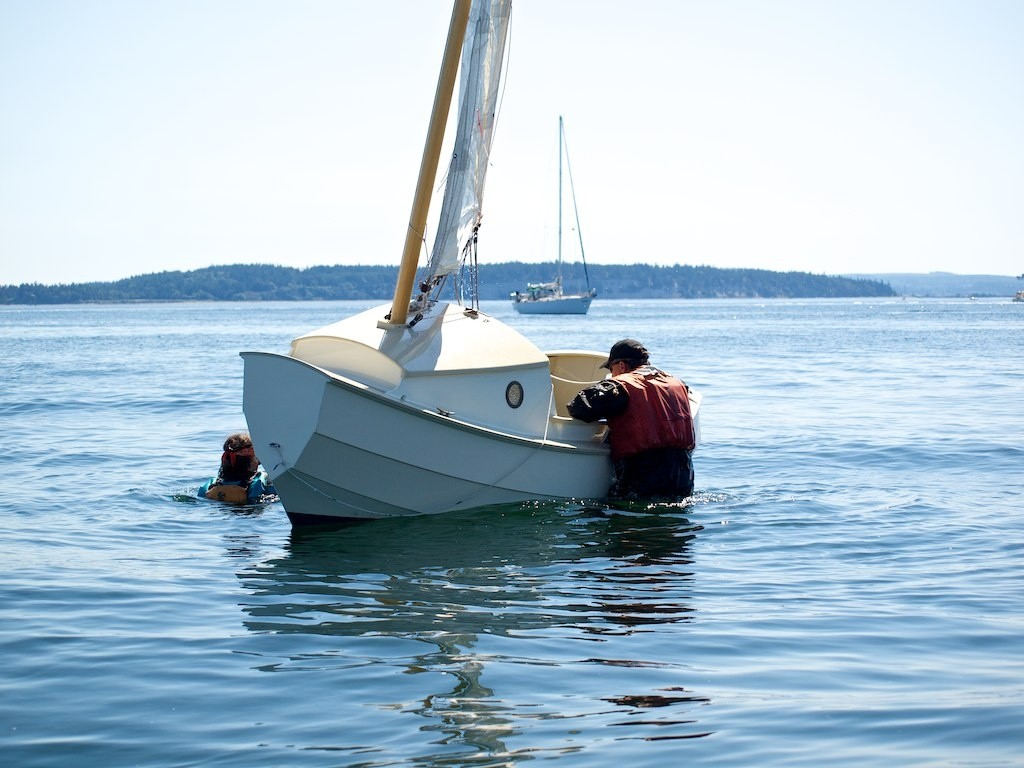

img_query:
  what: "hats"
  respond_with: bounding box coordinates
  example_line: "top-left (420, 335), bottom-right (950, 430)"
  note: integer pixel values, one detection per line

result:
top-left (599, 339), bottom-right (648, 369)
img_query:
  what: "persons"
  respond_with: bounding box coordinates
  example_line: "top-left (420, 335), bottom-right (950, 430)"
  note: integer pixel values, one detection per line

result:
top-left (198, 434), bottom-right (276, 500)
top-left (567, 339), bottom-right (696, 499)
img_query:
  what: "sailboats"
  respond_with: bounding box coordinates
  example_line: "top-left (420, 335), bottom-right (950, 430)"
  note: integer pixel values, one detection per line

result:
top-left (508, 117), bottom-right (606, 316)
top-left (241, 1), bottom-right (649, 523)
